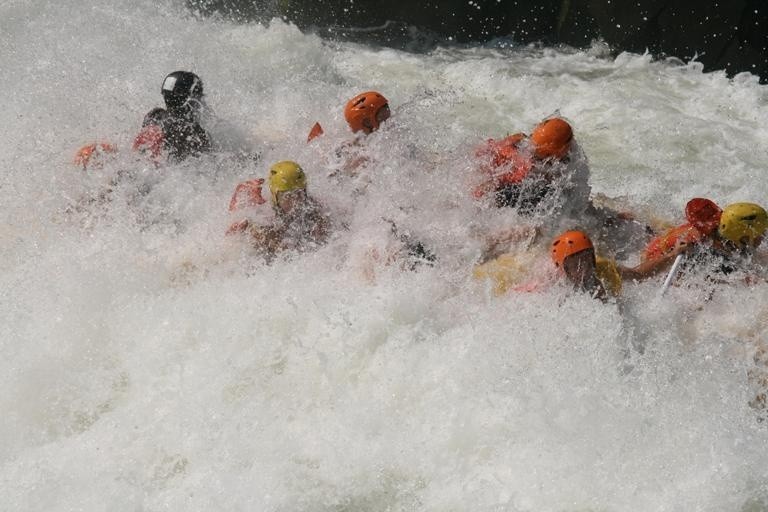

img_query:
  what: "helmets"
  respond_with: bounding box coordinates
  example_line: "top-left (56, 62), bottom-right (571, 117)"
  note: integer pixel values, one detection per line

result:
top-left (551, 231), bottom-right (597, 277)
top-left (531, 119), bottom-right (572, 158)
top-left (346, 91), bottom-right (389, 133)
top-left (160, 70), bottom-right (203, 113)
top-left (719, 203), bottom-right (767, 249)
top-left (267, 162), bottom-right (306, 207)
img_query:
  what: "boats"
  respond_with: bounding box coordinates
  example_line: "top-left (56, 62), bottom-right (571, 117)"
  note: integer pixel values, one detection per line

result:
top-left (111, 152), bottom-right (755, 382)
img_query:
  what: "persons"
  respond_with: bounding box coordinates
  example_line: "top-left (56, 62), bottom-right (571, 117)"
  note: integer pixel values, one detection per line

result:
top-left (226, 161), bottom-right (331, 266)
top-left (512, 231), bottom-right (688, 304)
top-left (132, 71), bottom-right (213, 165)
top-left (476, 118), bottom-right (573, 216)
top-left (344, 92), bottom-right (391, 136)
top-left (641, 203), bottom-right (768, 273)
